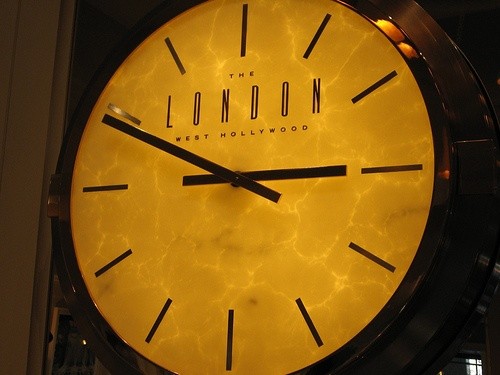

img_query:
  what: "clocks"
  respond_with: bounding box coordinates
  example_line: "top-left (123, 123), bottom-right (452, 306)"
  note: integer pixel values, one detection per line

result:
top-left (45, 0), bottom-right (499, 375)
